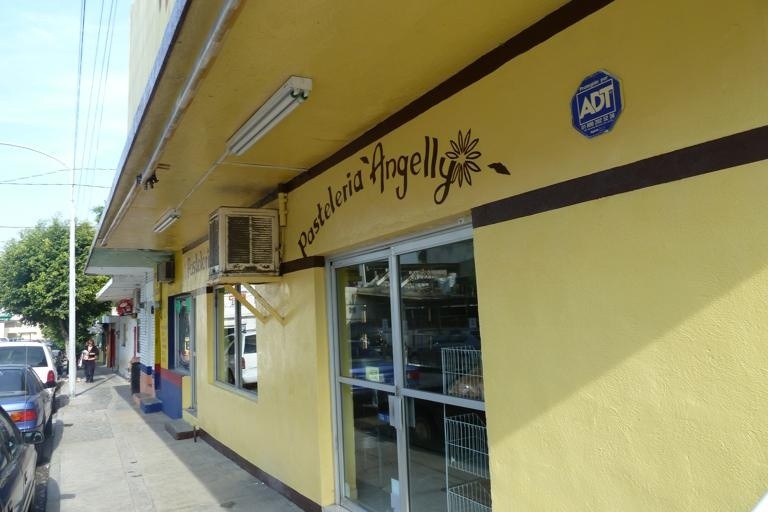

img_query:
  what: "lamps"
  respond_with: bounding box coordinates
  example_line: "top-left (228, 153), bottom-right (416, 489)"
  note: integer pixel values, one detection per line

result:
top-left (225, 76), bottom-right (312, 158)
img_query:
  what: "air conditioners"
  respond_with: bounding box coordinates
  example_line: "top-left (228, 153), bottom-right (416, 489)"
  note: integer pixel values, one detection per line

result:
top-left (157, 260), bottom-right (175, 280)
top-left (207, 205), bottom-right (280, 277)
top-left (152, 208), bottom-right (179, 232)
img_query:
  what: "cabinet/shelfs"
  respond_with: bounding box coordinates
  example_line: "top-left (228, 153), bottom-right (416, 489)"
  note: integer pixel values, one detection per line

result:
top-left (440, 346), bottom-right (495, 511)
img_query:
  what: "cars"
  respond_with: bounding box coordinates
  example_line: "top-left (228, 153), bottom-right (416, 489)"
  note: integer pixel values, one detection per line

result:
top-left (181, 337), bottom-right (190, 365)
top-left (224, 330), bottom-right (257, 387)
top-left (350, 324), bottom-right (484, 445)
top-left (0, 325), bottom-right (70, 511)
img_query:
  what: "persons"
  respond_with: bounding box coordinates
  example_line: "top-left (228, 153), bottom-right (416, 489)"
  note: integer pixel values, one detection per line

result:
top-left (83, 339), bottom-right (98, 383)
